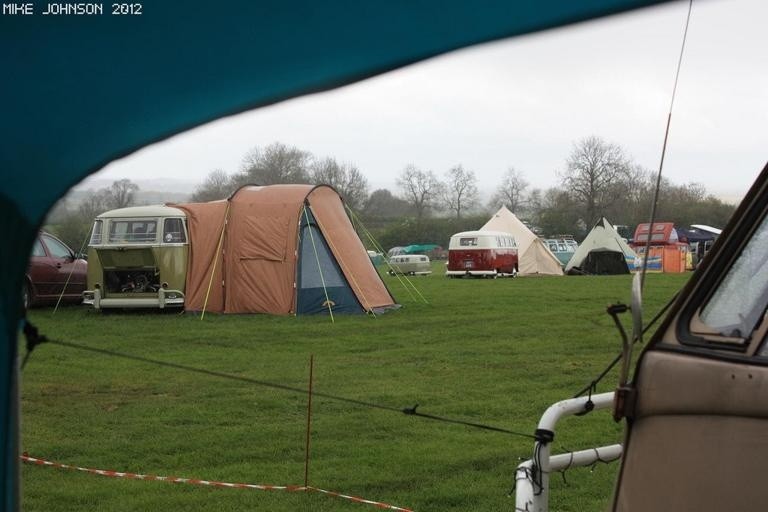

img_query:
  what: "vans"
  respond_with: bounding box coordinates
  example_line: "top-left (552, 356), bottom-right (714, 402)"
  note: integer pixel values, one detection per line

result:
top-left (388, 255), bottom-right (432, 276)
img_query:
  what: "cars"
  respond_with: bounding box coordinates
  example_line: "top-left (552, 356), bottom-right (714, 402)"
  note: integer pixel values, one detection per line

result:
top-left (22, 229), bottom-right (88, 309)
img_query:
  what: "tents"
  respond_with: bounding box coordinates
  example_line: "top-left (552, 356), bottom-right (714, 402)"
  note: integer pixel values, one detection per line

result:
top-left (163, 179), bottom-right (403, 316)
top-left (481, 205), bottom-right (564, 276)
top-left (567, 215), bottom-right (639, 275)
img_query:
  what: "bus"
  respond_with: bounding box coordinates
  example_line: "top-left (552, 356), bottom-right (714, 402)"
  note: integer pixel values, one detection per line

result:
top-left (82, 204), bottom-right (192, 310)
top-left (446, 231), bottom-right (519, 278)
